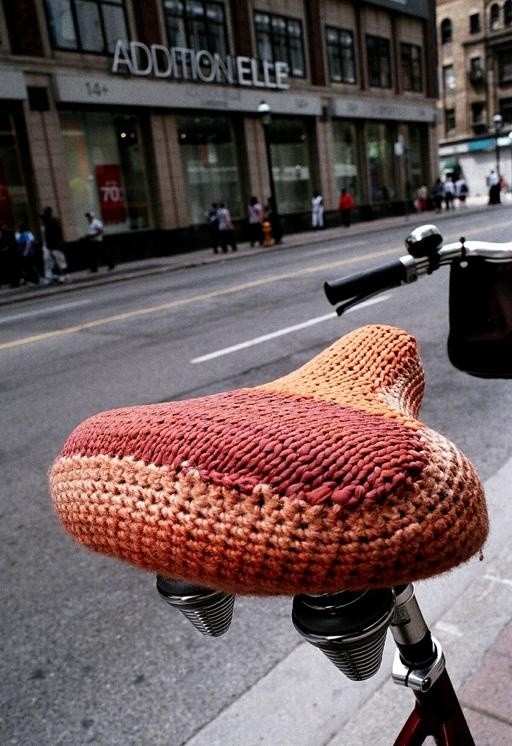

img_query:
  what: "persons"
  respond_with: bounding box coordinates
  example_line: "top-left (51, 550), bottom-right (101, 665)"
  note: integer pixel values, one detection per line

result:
top-left (456, 176), bottom-right (468, 210)
top-left (339, 188), bottom-right (354, 227)
top-left (40, 207), bottom-right (68, 285)
top-left (444, 177), bottom-right (455, 210)
top-left (415, 180), bottom-right (428, 213)
top-left (486, 170), bottom-right (501, 205)
top-left (14, 222), bottom-right (40, 287)
top-left (311, 190), bottom-right (325, 230)
top-left (208, 203), bottom-right (226, 254)
top-left (217, 204), bottom-right (236, 254)
top-left (433, 179), bottom-right (444, 213)
top-left (248, 197), bottom-right (264, 247)
top-left (84, 212), bottom-right (115, 273)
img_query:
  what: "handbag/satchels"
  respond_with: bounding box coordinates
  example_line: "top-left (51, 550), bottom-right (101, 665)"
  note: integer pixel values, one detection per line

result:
top-left (448, 258), bottom-right (511, 378)
top-left (227, 224), bottom-right (234, 231)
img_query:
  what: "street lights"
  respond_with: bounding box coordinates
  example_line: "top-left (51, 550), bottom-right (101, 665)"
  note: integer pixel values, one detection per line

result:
top-left (257, 101), bottom-right (285, 245)
top-left (492, 114), bottom-right (502, 201)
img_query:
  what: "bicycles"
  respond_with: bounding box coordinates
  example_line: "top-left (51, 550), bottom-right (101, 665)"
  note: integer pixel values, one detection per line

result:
top-left (45, 214), bottom-right (512, 746)
